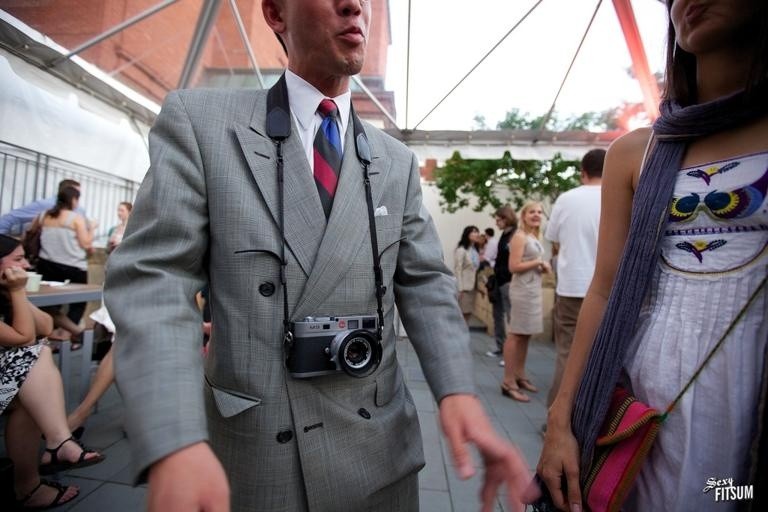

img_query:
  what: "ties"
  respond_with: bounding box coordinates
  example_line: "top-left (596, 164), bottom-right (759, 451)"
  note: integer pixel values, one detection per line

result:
top-left (310, 97), bottom-right (344, 222)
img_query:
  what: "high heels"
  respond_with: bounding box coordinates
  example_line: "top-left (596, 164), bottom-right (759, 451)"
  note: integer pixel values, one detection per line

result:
top-left (499, 382), bottom-right (530, 404)
top-left (515, 378), bottom-right (538, 393)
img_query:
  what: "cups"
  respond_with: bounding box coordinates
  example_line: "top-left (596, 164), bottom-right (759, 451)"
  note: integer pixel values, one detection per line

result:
top-left (28, 273), bottom-right (43, 291)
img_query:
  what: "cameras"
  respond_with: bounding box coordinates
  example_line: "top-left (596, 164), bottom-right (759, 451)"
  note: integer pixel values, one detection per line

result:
top-left (288, 315), bottom-right (383, 378)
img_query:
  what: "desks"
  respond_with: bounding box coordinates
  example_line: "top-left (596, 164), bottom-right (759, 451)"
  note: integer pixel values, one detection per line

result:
top-left (25, 280), bottom-right (104, 415)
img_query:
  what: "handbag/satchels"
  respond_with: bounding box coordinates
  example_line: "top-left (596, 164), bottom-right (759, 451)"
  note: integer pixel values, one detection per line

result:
top-left (580, 342), bottom-right (702, 512)
top-left (484, 271), bottom-right (508, 304)
top-left (23, 211), bottom-right (44, 262)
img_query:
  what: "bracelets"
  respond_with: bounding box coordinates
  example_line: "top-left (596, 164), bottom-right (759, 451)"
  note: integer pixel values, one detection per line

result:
top-left (539, 259), bottom-right (544, 268)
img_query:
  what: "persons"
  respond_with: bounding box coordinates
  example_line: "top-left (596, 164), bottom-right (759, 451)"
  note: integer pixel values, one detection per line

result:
top-left (482, 227), bottom-right (498, 268)
top-left (108, 200), bottom-right (131, 241)
top-left (476, 233), bottom-right (486, 253)
top-left (43, 346), bottom-right (118, 442)
top-left (522, 0), bottom-right (768, 511)
top-left (452, 226), bottom-right (479, 322)
top-left (545, 148), bottom-right (608, 408)
top-left (0, 179), bottom-right (88, 236)
top-left (29, 187), bottom-right (95, 352)
top-left (103, 1), bottom-right (531, 512)
top-left (0, 233), bottom-right (106, 510)
top-left (501, 202), bottom-right (552, 403)
top-left (485, 207), bottom-right (518, 358)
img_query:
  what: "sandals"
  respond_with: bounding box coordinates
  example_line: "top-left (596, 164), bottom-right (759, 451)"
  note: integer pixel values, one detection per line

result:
top-left (40, 439), bottom-right (106, 473)
top-left (13, 482), bottom-right (81, 509)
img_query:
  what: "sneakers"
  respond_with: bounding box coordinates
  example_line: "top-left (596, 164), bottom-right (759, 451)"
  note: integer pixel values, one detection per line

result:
top-left (67, 339), bottom-right (84, 351)
top-left (485, 345), bottom-right (501, 358)
